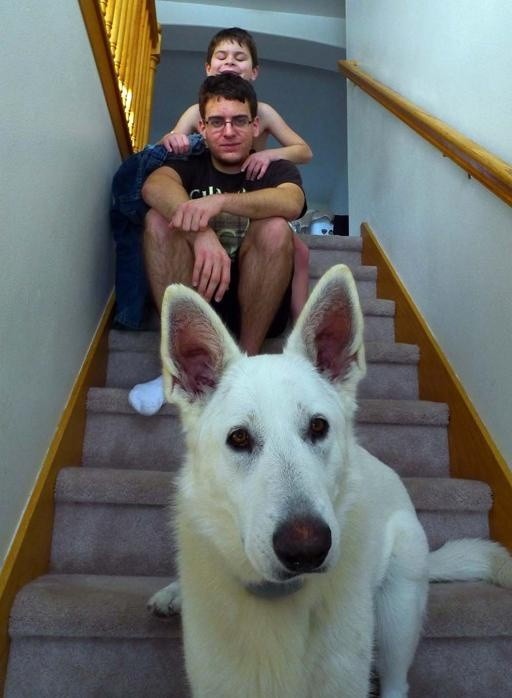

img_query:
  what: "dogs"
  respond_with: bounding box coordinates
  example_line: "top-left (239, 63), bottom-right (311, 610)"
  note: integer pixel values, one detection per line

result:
top-left (140, 263), bottom-right (511, 698)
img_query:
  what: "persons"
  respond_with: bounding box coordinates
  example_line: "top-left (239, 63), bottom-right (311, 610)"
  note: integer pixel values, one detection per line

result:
top-left (127, 71), bottom-right (308, 418)
top-left (151, 27), bottom-right (315, 182)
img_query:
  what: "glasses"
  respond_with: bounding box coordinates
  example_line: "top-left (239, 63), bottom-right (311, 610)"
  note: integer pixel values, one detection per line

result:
top-left (205, 117), bottom-right (254, 129)
